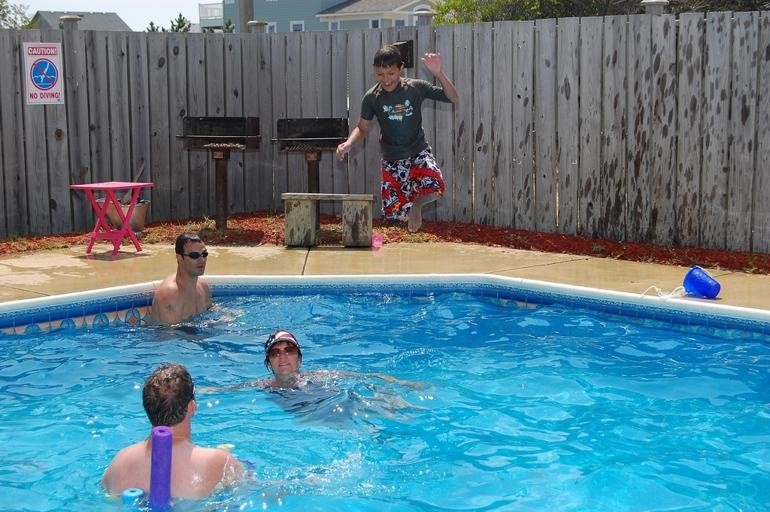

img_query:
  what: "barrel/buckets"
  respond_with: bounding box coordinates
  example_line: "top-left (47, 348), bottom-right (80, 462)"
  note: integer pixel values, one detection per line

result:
top-left (108, 200), bottom-right (150, 228)
top-left (683, 264), bottom-right (720, 299)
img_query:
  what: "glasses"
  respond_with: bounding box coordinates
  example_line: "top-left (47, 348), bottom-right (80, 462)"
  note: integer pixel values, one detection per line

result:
top-left (269, 347), bottom-right (296, 357)
top-left (182, 252), bottom-right (208, 259)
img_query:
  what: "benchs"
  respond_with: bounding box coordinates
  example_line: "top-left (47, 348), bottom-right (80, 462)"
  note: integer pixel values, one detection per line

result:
top-left (284, 192), bottom-right (374, 247)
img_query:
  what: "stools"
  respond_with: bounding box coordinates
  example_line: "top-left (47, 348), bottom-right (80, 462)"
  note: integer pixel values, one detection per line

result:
top-left (71, 178), bottom-right (154, 258)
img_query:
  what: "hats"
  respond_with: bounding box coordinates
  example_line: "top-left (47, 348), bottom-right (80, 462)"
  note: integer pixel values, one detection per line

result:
top-left (265, 330), bottom-right (300, 352)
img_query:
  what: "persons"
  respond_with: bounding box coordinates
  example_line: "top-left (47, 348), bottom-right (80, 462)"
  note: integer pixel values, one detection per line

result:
top-left (336, 44), bottom-right (459, 232)
top-left (150, 233), bottom-right (243, 331)
